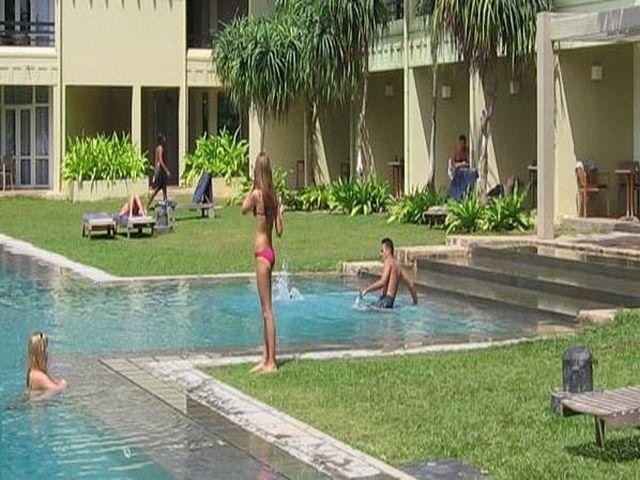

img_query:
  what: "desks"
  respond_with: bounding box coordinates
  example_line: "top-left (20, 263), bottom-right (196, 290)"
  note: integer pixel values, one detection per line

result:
top-left (613, 168), bottom-right (640, 222)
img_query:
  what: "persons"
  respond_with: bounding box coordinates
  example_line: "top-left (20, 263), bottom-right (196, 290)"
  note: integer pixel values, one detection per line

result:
top-left (242, 153), bottom-right (287, 374)
top-left (451, 134), bottom-right (467, 164)
top-left (121, 194), bottom-right (145, 218)
top-left (26, 332), bottom-right (69, 391)
top-left (361, 237), bottom-right (417, 312)
top-left (147, 132), bottom-right (171, 208)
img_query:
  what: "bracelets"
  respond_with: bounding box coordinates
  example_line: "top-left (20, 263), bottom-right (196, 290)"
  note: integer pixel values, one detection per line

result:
top-left (366, 289), bottom-right (368, 292)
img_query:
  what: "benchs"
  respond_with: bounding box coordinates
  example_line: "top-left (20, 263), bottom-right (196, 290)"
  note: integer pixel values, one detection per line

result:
top-left (565, 386), bottom-right (640, 448)
top-left (81, 211), bottom-right (157, 239)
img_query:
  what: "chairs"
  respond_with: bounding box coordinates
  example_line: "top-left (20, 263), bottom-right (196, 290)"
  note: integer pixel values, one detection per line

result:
top-left (576, 161), bottom-right (611, 217)
top-left (421, 166), bottom-right (478, 230)
